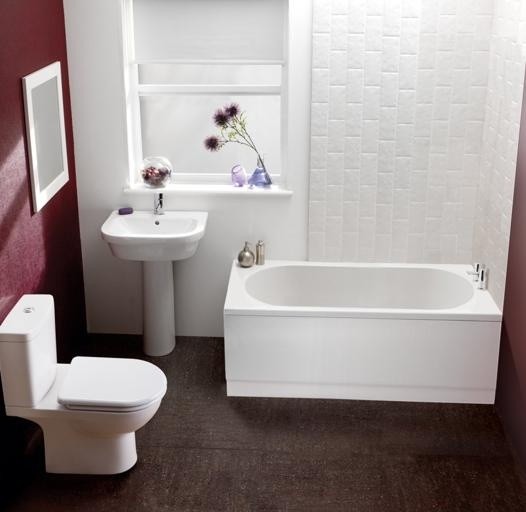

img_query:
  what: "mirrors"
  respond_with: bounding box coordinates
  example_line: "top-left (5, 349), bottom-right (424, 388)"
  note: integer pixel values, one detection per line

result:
top-left (18, 60), bottom-right (70, 214)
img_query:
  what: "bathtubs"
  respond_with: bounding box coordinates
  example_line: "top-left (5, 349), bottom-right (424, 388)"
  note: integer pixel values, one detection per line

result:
top-left (222, 258), bottom-right (504, 404)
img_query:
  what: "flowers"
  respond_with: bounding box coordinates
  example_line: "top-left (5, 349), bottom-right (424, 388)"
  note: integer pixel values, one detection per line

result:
top-left (203, 102), bottom-right (271, 182)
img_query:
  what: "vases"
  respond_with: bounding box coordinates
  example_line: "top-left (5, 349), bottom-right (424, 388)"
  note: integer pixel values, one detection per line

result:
top-left (248, 151), bottom-right (271, 186)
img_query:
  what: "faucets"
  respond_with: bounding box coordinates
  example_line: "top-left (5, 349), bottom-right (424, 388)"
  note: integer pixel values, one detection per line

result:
top-left (465, 262), bottom-right (481, 281)
top-left (153, 192), bottom-right (165, 215)
top-left (474, 267), bottom-right (489, 290)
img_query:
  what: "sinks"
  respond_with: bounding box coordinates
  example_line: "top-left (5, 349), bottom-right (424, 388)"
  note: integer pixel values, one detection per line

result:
top-left (100, 209), bottom-right (209, 262)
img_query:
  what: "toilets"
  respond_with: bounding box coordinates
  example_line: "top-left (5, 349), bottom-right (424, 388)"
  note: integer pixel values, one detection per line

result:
top-left (0, 294), bottom-right (168, 474)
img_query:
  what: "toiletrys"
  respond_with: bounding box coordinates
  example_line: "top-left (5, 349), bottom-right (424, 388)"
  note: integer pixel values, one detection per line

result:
top-left (238, 240), bottom-right (265, 267)
